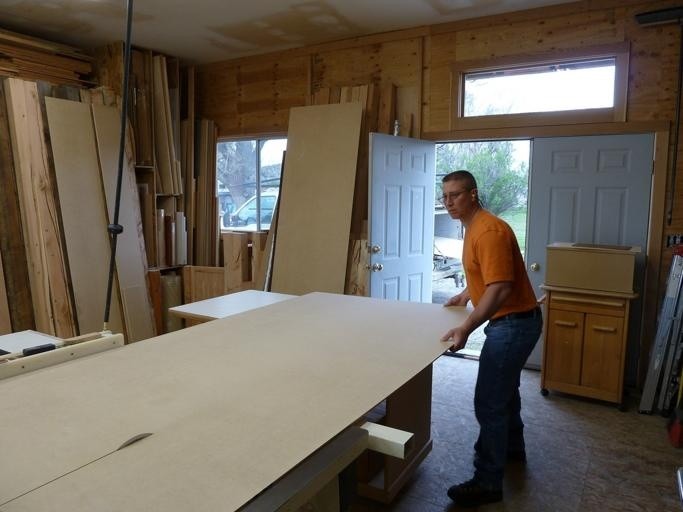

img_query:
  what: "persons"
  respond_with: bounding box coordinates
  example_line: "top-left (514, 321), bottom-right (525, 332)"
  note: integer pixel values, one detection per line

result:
top-left (438, 169), bottom-right (544, 506)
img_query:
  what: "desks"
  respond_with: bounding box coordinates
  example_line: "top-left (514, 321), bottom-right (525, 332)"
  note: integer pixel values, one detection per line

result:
top-left (168, 288), bottom-right (433, 505)
top-left (234, 426), bottom-right (368, 510)
top-left (0, 330), bottom-right (112, 364)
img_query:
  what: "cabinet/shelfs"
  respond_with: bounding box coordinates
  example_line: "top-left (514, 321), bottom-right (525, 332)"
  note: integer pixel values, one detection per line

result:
top-left (536, 282), bottom-right (639, 412)
top-left (98, 40), bottom-right (193, 270)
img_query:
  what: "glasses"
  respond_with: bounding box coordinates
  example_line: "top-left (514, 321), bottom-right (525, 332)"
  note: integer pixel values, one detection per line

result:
top-left (438, 191), bottom-right (466, 203)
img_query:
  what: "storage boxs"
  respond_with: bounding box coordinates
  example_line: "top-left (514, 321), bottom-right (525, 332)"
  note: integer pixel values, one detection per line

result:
top-left (543, 242), bottom-right (642, 294)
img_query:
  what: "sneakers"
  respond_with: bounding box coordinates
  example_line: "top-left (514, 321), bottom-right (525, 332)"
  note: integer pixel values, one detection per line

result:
top-left (447, 476), bottom-right (503, 505)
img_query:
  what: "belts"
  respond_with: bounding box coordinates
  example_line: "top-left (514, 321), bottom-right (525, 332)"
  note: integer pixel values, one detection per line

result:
top-left (493, 306), bottom-right (540, 320)
